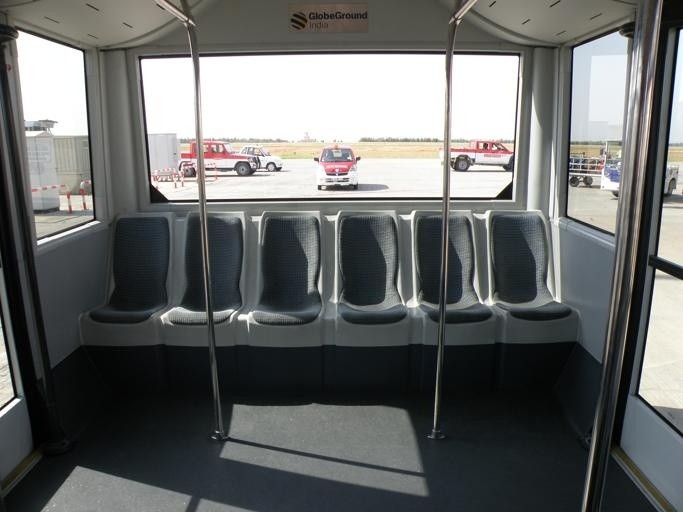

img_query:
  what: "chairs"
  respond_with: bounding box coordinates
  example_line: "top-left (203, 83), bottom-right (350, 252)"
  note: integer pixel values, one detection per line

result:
top-left (160, 211), bottom-right (252, 347)
top-left (411, 211), bottom-right (498, 345)
top-left (322, 209), bottom-right (411, 347)
top-left (237, 211), bottom-right (326, 346)
top-left (484, 210), bottom-right (580, 345)
top-left (80, 212), bottom-right (177, 347)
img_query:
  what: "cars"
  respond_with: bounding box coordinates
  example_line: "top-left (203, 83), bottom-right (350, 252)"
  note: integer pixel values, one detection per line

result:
top-left (314, 145), bottom-right (361, 190)
top-left (239, 144), bottom-right (283, 172)
top-left (601, 138), bottom-right (679, 197)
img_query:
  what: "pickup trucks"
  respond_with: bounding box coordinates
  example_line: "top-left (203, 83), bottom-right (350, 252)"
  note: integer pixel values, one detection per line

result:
top-left (180, 140), bottom-right (261, 177)
top-left (439, 140), bottom-right (514, 172)
